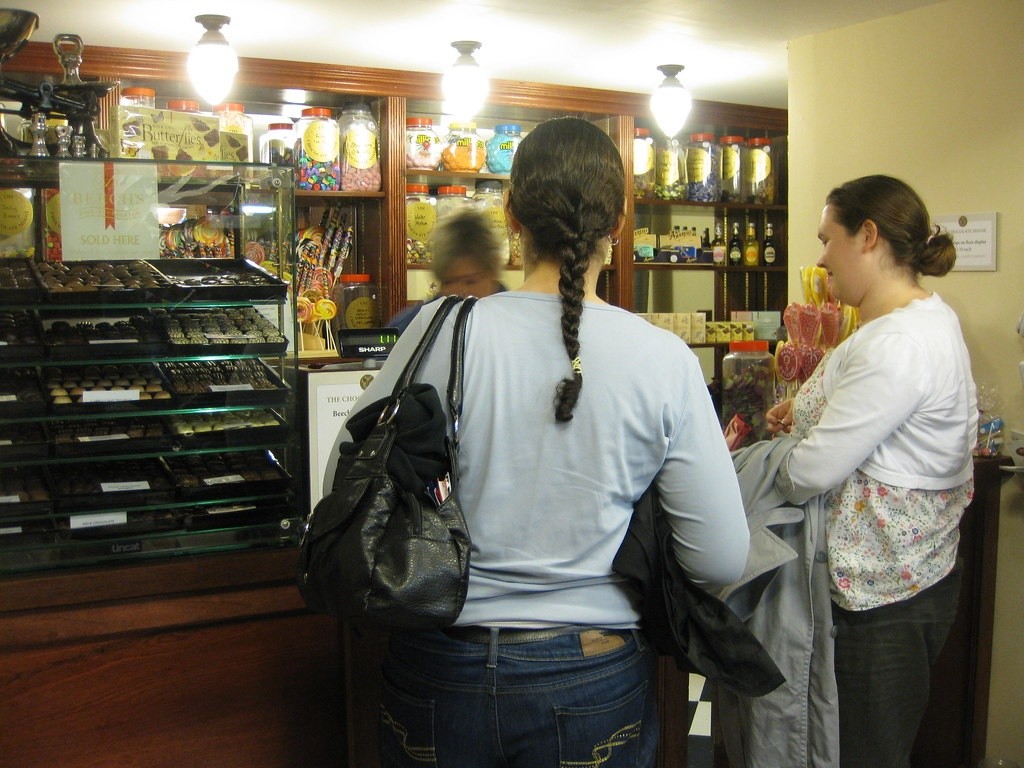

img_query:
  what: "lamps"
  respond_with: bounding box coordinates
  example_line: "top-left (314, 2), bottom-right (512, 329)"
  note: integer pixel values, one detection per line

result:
top-left (649, 63), bottom-right (692, 139)
top-left (443, 36), bottom-right (486, 119)
top-left (186, 2), bottom-right (240, 105)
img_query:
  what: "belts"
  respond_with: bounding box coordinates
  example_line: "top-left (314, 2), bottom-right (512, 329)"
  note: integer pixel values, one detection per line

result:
top-left (442, 625), bottom-right (600, 645)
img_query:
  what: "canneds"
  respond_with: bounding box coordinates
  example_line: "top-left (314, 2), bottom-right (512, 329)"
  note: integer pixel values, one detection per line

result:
top-left (720, 340), bottom-right (777, 449)
top-left (119, 86), bottom-right (254, 163)
top-left (406, 118), bottom-right (524, 266)
top-left (335, 274), bottom-right (379, 329)
top-left (632, 127), bottom-right (778, 204)
top-left (258, 104), bottom-right (382, 191)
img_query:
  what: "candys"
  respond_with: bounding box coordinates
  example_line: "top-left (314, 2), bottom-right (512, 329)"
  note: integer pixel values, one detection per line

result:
top-left (773, 265), bottom-right (861, 383)
top-left (973, 441), bottom-right (999, 456)
top-left (159, 216), bottom-right (337, 323)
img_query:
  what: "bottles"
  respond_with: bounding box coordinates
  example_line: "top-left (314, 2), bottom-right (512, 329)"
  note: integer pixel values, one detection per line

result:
top-left (722, 340), bottom-right (776, 451)
top-left (629, 126), bottom-right (779, 266)
top-left (332, 274), bottom-right (379, 354)
top-left (123, 88), bottom-right (383, 192)
top-left (404, 119), bottom-right (525, 268)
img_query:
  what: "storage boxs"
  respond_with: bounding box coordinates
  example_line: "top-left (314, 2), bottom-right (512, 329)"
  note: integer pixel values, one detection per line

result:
top-left (631, 309), bottom-right (782, 344)
top-left (219, 131), bottom-right (249, 162)
top-left (107, 106), bottom-right (221, 162)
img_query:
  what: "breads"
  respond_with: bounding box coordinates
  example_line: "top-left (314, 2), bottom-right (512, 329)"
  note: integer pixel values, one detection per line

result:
top-left (0, 359), bottom-right (278, 404)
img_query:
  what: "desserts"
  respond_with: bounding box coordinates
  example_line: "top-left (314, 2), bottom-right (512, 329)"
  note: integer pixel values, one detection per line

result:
top-left (0, 451), bottom-right (283, 532)
top-left (0, 261), bottom-right (270, 292)
top-left (0, 409), bottom-right (281, 444)
top-left (0, 308), bottom-right (286, 344)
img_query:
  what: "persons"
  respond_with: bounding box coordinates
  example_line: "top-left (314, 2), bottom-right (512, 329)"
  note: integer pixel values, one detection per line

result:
top-left (322, 117), bottom-right (747, 768)
top-left (380, 215), bottom-right (511, 337)
top-left (732, 174), bottom-right (982, 768)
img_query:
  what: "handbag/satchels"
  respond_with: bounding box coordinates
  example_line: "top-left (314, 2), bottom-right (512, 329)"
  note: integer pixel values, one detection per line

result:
top-left (291, 292), bottom-right (475, 631)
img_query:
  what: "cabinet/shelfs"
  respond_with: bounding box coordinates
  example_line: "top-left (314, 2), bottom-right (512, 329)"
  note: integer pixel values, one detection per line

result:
top-left (628, 93), bottom-right (789, 314)
top-left (0, 36), bottom-right (628, 362)
